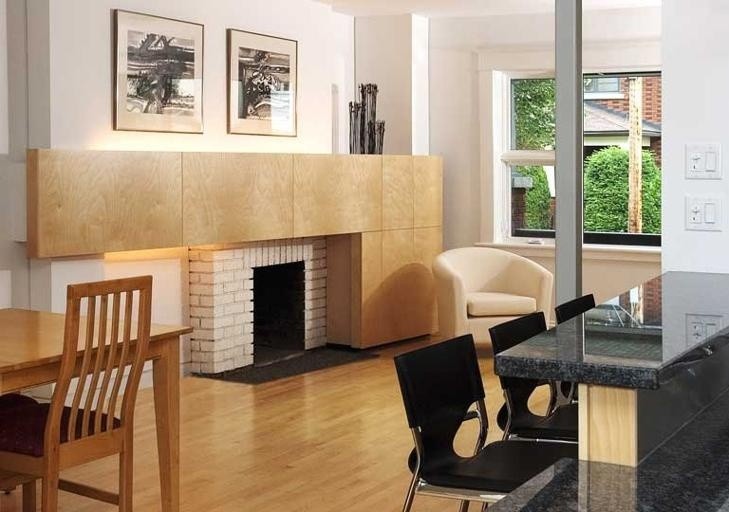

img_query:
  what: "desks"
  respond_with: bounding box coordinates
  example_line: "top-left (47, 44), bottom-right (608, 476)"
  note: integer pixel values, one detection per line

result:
top-left (0, 306), bottom-right (194, 512)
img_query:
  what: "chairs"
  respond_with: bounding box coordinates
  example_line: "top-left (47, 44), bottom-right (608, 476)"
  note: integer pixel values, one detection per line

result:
top-left (431, 246), bottom-right (554, 354)
top-left (392, 333), bottom-right (568, 512)
top-left (1, 273), bottom-right (154, 512)
top-left (488, 310), bottom-right (578, 444)
top-left (554, 293), bottom-right (596, 401)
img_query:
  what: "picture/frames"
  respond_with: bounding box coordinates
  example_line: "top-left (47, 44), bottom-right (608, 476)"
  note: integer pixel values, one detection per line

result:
top-left (113, 7), bottom-right (300, 139)
